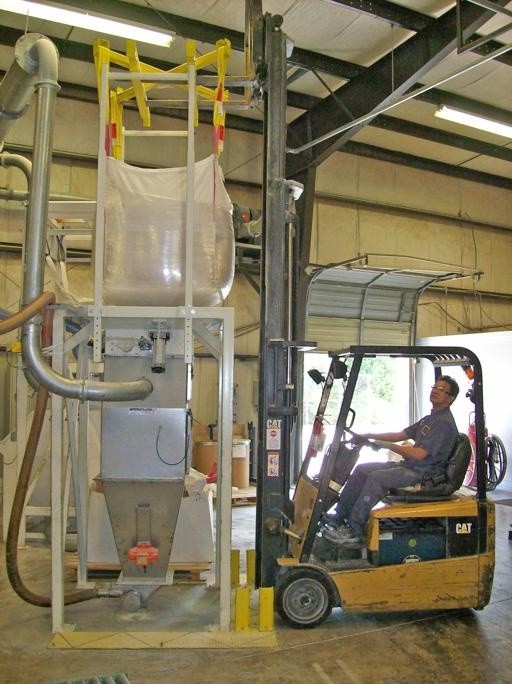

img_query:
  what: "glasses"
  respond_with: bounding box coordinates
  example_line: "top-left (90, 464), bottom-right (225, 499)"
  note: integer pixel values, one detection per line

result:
top-left (431, 385), bottom-right (452, 395)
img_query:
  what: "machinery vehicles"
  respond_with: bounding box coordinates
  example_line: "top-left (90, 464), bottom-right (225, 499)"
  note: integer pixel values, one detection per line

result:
top-left (113, 8), bottom-right (501, 629)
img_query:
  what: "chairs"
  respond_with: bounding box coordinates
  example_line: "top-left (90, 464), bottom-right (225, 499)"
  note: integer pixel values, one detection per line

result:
top-left (381, 432), bottom-right (473, 504)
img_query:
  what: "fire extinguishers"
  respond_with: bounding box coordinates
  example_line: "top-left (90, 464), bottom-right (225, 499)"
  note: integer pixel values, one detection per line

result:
top-left (306, 414), bottom-right (331, 457)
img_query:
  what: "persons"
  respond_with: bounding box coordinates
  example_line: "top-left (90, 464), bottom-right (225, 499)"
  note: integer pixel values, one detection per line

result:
top-left (317, 374), bottom-right (459, 546)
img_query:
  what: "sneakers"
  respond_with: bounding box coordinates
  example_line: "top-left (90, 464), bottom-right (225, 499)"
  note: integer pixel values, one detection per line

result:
top-left (322, 524), bottom-right (360, 543)
top-left (324, 513), bottom-right (346, 528)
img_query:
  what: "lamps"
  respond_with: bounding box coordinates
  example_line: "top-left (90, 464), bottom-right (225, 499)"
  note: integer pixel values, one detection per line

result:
top-left (1, 0), bottom-right (177, 49)
top-left (432, 103), bottom-right (511, 138)
